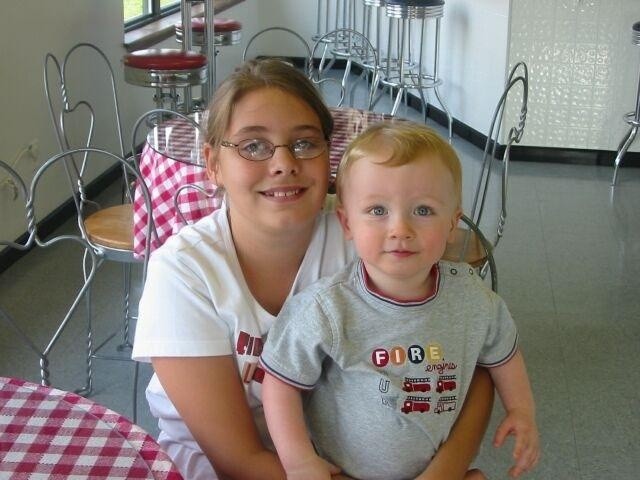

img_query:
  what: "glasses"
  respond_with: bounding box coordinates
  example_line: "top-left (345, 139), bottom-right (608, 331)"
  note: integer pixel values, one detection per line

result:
top-left (205, 135), bottom-right (333, 162)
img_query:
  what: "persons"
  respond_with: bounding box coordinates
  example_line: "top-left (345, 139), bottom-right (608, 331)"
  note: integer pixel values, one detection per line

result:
top-left (128, 58), bottom-right (496, 480)
top-left (258, 120), bottom-right (539, 480)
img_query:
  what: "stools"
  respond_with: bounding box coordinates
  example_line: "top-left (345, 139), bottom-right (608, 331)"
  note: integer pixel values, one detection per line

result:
top-left (123, 17), bottom-right (242, 204)
top-left (312, 0), bottom-right (454, 143)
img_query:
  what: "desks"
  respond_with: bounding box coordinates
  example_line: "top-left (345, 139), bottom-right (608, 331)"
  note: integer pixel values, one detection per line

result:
top-left (127, 106), bottom-right (427, 267)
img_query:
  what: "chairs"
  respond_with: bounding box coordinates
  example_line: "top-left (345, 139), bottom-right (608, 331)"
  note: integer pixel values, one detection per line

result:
top-left (439, 60), bottom-right (527, 295)
top-left (242, 28), bottom-right (347, 107)
top-left (3, 147), bottom-right (153, 424)
top-left (44, 41), bottom-right (145, 398)
top-left (130, 109), bottom-right (220, 286)
